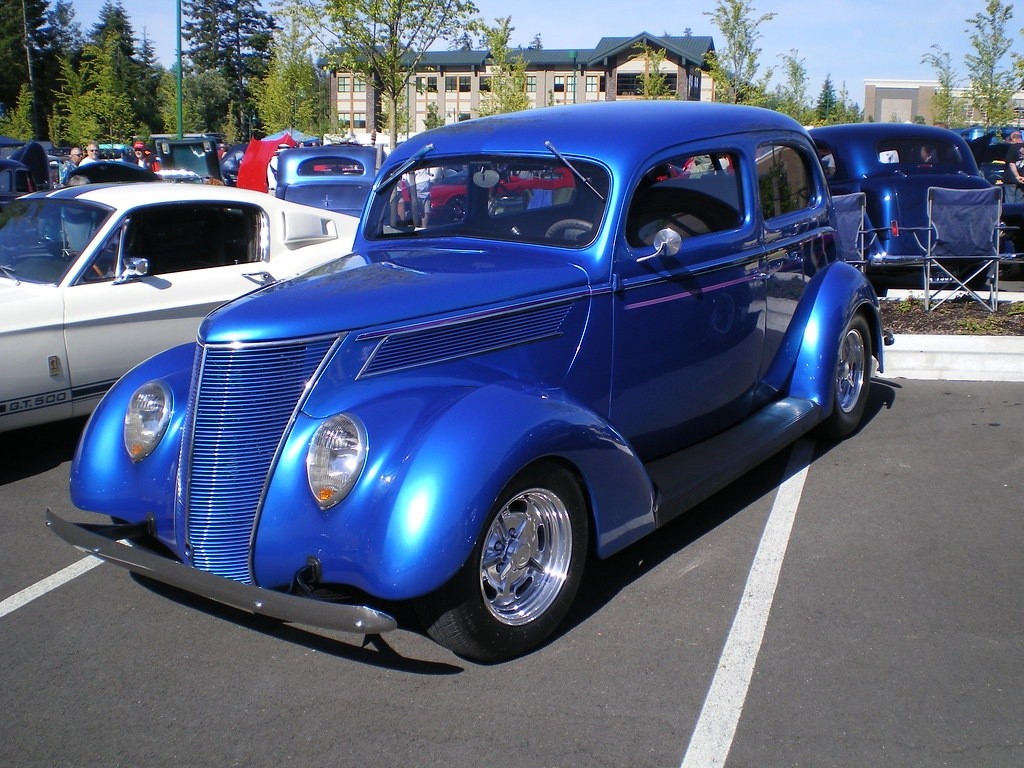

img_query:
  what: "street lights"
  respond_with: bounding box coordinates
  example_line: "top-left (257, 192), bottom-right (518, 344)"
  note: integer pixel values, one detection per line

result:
top-left (404, 59), bottom-right (409, 141)
top-left (445, 108), bottom-right (465, 123)
top-left (569, 50), bottom-right (578, 104)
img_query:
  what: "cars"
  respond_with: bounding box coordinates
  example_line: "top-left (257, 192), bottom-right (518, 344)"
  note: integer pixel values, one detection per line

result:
top-left (0, 122), bottom-right (697, 231)
top-left (754, 121), bottom-right (1024, 296)
top-left (0, 181), bottom-right (408, 435)
top-left (44, 98), bottom-right (895, 666)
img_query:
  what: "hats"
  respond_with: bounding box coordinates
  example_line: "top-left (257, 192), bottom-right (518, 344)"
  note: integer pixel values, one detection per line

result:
top-left (134, 142), bottom-right (145, 150)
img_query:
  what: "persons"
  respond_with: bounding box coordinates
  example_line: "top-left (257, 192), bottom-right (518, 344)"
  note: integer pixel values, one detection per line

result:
top-left (389, 167), bottom-right (432, 229)
top-left (59, 141), bottom-right (318, 254)
top-left (821, 121), bottom-right (1024, 204)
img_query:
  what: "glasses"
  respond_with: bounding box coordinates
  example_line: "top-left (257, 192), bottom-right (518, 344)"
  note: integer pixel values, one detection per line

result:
top-left (90, 150), bottom-right (99, 152)
top-left (74, 154), bottom-right (83, 156)
